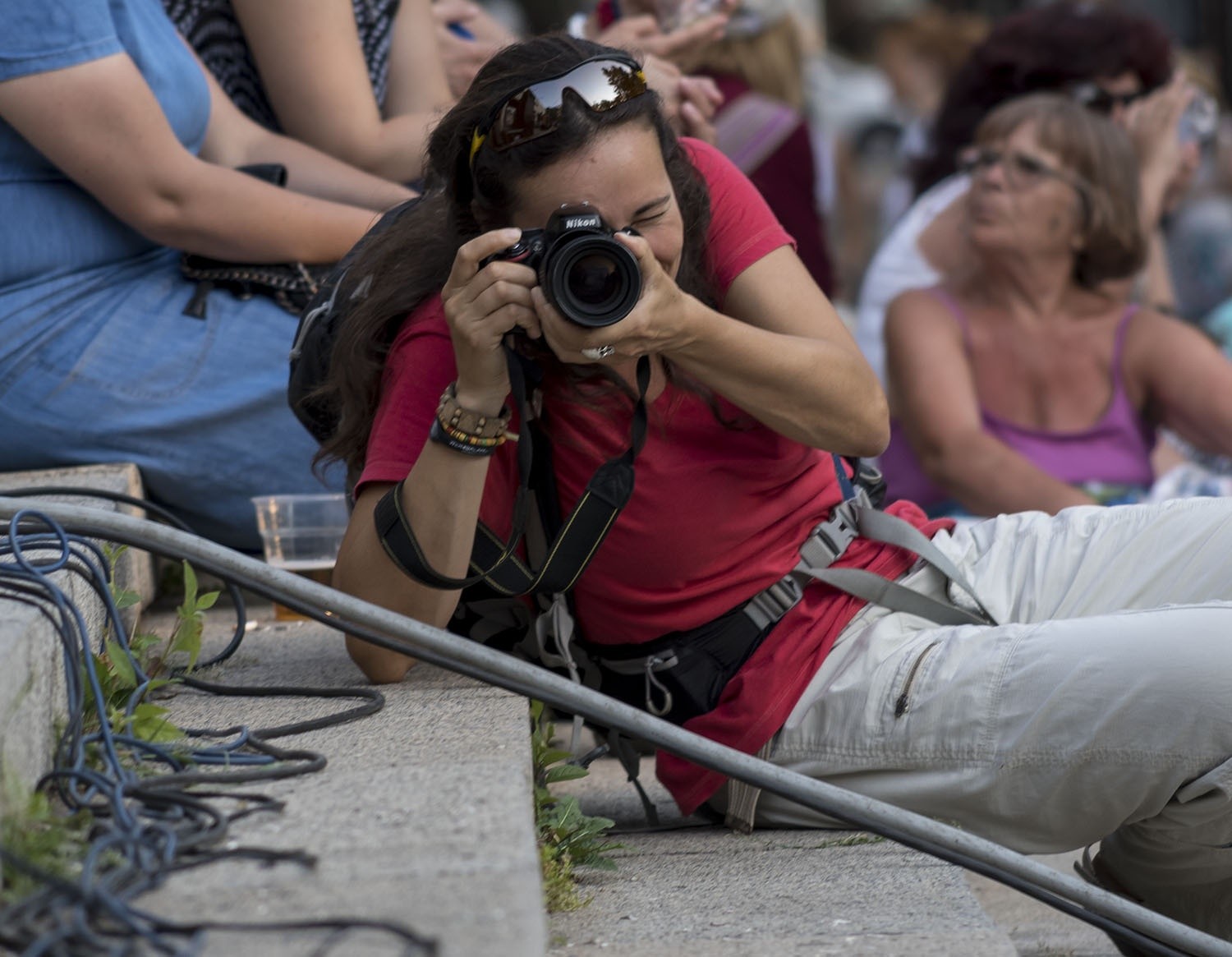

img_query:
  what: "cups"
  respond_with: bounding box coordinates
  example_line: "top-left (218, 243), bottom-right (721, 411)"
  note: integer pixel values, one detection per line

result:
top-left (252, 493), bottom-right (350, 621)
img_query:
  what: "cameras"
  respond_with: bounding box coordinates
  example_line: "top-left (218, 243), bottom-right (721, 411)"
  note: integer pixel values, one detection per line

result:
top-left (492, 204), bottom-right (643, 331)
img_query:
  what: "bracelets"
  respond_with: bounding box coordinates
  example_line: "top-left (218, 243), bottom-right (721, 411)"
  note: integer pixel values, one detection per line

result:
top-left (433, 407), bottom-right (508, 447)
top-left (438, 382), bottom-right (512, 438)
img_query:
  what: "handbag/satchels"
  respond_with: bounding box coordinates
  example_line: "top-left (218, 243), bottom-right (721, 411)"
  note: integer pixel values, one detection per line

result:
top-left (180, 162), bottom-right (326, 316)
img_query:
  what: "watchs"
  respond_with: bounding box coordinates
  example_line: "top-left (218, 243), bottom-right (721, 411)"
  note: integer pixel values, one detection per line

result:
top-left (429, 419), bottom-right (505, 456)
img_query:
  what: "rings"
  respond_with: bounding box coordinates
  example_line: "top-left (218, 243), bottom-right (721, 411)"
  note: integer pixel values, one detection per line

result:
top-left (580, 345), bottom-right (616, 360)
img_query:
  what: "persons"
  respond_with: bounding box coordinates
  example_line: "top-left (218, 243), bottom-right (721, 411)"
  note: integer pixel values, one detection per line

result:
top-left (296, 32), bottom-right (1232, 944)
top-left (164, 1), bottom-right (1232, 526)
top-left (0, 2), bottom-right (440, 564)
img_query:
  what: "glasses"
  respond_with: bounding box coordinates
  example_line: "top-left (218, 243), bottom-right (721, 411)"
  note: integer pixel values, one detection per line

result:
top-left (960, 146), bottom-right (1091, 229)
top-left (1074, 82), bottom-right (1148, 115)
top-left (469, 55), bottom-right (648, 167)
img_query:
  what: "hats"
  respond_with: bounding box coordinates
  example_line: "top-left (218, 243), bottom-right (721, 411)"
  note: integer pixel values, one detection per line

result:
top-left (930, 12), bottom-right (1172, 173)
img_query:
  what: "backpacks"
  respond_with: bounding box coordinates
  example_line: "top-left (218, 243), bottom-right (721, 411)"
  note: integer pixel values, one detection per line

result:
top-left (286, 192), bottom-right (576, 623)
